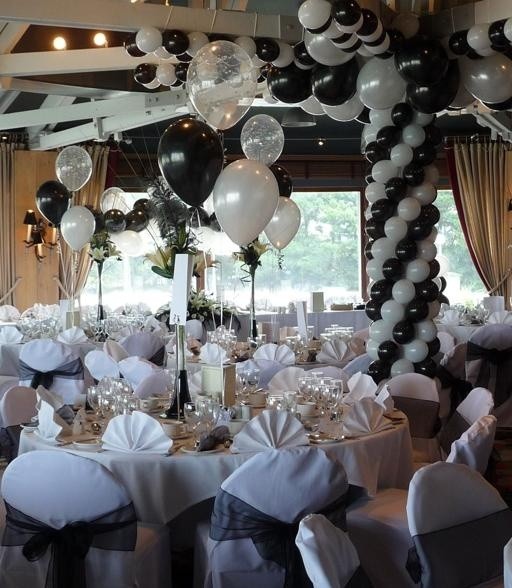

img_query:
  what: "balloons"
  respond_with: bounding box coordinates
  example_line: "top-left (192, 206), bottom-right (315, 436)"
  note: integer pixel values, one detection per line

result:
top-left (333, 13), bottom-right (364, 34)
top-left (382, 258), bottom-right (404, 282)
top-left (408, 219), bottom-right (432, 241)
top-left (293, 41), bottom-right (320, 66)
top-left (332, 0), bottom-right (362, 27)
top-left (186, 31), bottom-right (209, 58)
top-left (365, 258), bottom-right (386, 281)
top-left (393, 16), bottom-right (512, 113)
top-left (366, 338), bottom-right (382, 361)
top-left (413, 279), bottom-right (439, 303)
top-left (234, 35), bottom-right (258, 61)
top-left (392, 278), bottom-right (416, 305)
top-left (99, 186), bottom-right (130, 215)
top-left (263, 196), bottom-right (301, 251)
top-left (421, 204), bottom-right (441, 226)
top-left (397, 197), bottom-right (422, 222)
top-left (122, 32), bottom-right (148, 58)
top-left (364, 240), bottom-right (375, 260)
top-left (322, 22), bottom-right (345, 39)
top-left (426, 299), bottom-right (441, 321)
top-left (132, 198), bottom-right (158, 220)
top-left (331, 36), bottom-right (358, 50)
top-left (60, 204), bottom-right (96, 253)
top-left (212, 158), bottom-right (280, 248)
top-left (239, 113), bottom-right (286, 168)
top-left (426, 226), bottom-right (438, 243)
top-left (428, 259), bottom-right (440, 280)
top-left (320, 91), bottom-right (365, 123)
top-left (383, 216), bottom-right (408, 245)
top-left (414, 358), bottom-right (437, 379)
top-left (440, 276), bottom-right (446, 293)
top-left (269, 164), bottom-right (294, 198)
top-left (272, 42), bottom-right (295, 68)
top-left (431, 277), bottom-right (442, 292)
top-left (35, 180), bottom-right (69, 228)
top-left (371, 198), bottom-right (395, 224)
top-left (186, 39), bottom-right (258, 131)
top-left (374, 40), bottom-right (397, 59)
top-left (367, 360), bottom-right (391, 385)
top-left (303, 31), bottom-right (357, 67)
top-left (311, 64), bottom-right (361, 107)
top-left (365, 300), bottom-right (383, 321)
top-left (303, 11), bottom-right (333, 35)
top-left (261, 88), bottom-right (281, 108)
top-left (386, 28), bottom-right (404, 54)
top-left (403, 339), bottom-right (429, 362)
top-left (254, 37), bottom-right (280, 63)
top-left (364, 204), bottom-right (374, 221)
top-left (378, 340), bottom-right (400, 366)
top-left (356, 57), bottom-right (408, 111)
top-left (363, 102), bottom-right (443, 203)
top-left (133, 62), bottom-right (190, 92)
top-left (365, 217), bottom-right (387, 240)
top-left (413, 319), bottom-right (438, 343)
top-left (125, 209), bottom-right (148, 233)
top-left (340, 39), bottom-right (363, 54)
top-left (156, 117), bottom-right (224, 208)
top-left (426, 336), bottom-right (441, 359)
top-left (370, 279), bottom-right (395, 306)
top-left (161, 28), bottom-right (190, 56)
top-left (380, 299), bottom-right (406, 324)
top-left (405, 258), bottom-right (431, 283)
top-left (390, 358), bottom-right (415, 378)
top-left (392, 321), bottom-right (416, 345)
top-left (330, 32), bottom-right (352, 43)
top-left (395, 238), bottom-right (417, 263)
top-left (370, 236), bottom-right (396, 261)
top-left (354, 106), bottom-right (372, 127)
top-left (266, 62), bottom-right (313, 104)
top-left (54, 145), bottom-right (93, 195)
top-left (299, 95), bottom-right (326, 116)
top-left (291, 57), bottom-right (317, 71)
top-left (369, 318), bottom-right (395, 344)
top-left (356, 7), bottom-right (396, 59)
top-left (416, 240), bottom-right (438, 262)
top-left (406, 299), bottom-right (429, 324)
top-left (297, 0), bottom-right (333, 30)
top-left (135, 25), bottom-right (163, 54)
top-left (103, 209), bottom-right (127, 235)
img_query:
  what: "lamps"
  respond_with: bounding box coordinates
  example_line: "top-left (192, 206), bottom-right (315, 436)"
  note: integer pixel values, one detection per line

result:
top-left (22, 209), bottom-right (57, 263)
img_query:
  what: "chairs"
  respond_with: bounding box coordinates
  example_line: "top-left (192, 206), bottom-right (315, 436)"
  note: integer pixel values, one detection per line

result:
top-left (345, 416), bottom-right (497, 588)
top-left (407, 462), bottom-right (512, 588)
top-left (193, 447), bottom-right (348, 588)
top-left (1, 450), bottom-right (172, 588)
top-left (119, 332), bottom-right (165, 378)
top-left (465, 325), bottom-right (512, 427)
top-left (239, 358), bottom-right (284, 390)
top-left (295, 514), bottom-right (373, 588)
top-left (19, 338), bottom-right (84, 406)
top-left (438, 341), bottom-right (466, 383)
top-left (436, 331), bottom-right (455, 355)
top-left (377, 372), bottom-right (440, 463)
top-left (0, 386), bottom-right (38, 464)
top-left (410, 387), bottom-right (494, 469)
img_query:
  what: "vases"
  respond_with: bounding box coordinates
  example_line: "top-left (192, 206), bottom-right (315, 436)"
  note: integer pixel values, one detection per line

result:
top-left (97, 305), bottom-right (103, 321)
top-left (253, 320), bottom-right (257, 338)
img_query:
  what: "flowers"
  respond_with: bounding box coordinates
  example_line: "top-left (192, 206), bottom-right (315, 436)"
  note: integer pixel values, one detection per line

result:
top-left (84, 232), bottom-right (123, 305)
top-left (232, 236), bottom-right (273, 319)
top-left (156, 296), bottom-right (241, 332)
top-left (143, 176), bottom-right (221, 280)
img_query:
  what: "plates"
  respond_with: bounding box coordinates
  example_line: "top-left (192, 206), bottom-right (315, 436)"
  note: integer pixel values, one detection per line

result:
top-left (302, 414), bottom-right (318, 417)
top-left (170, 434), bottom-right (188, 440)
top-left (74, 439), bottom-right (101, 446)
top-left (252, 405), bottom-right (266, 408)
top-left (181, 444), bottom-right (223, 454)
top-left (309, 432), bottom-right (344, 444)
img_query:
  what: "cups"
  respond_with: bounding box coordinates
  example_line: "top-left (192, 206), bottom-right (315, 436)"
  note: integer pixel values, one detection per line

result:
top-left (297, 401), bottom-right (315, 414)
top-left (254, 391), bottom-right (269, 405)
top-left (141, 399), bottom-right (153, 413)
top-left (226, 420), bottom-right (248, 440)
top-left (162, 420), bottom-right (183, 436)
top-left (444, 311), bottom-right (458, 326)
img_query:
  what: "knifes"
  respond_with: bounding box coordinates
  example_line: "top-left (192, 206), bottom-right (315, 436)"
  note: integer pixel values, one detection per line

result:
top-left (253, 388), bottom-right (263, 393)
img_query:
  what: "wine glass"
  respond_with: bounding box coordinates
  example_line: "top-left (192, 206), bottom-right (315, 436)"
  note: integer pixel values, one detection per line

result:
top-left (257, 333), bottom-right (267, 348)
top-left (313, 385), bottom-right (329, 417)
top-left (267, 392), bottom-right (297, 415)
top-left (89, 309), bottom-right (145, 342)
top-left (207, 325), bottom-right (237, 356)
top-left (248, 337), bottom-right (256, 354)
top-left (164, 368), bottom-right (175, 397)
top-left (297, 377), bottom-right (313, 400)
top-left (320, 324), bottom-right (354, 345)
top-left (328, 385), bottom-right (340, 423)
top-left (160, 398), bottom-right (171, 418)
top-left (184, 398), bottom-right (233, 446)
top-left (321, 377), bottom-right (332, 388)
top-left (311, 372), bottom-right (324, 386)
top-left (247, 369), bottom-right (260, 387)
top-left (330, 379), bottom-right (343, 402)
top-left (88, 377), bottom-right (140, 420)
top-left (285, 332), bottom-right (312, 362)
top-left (234, 369), bottom-right (246, 388)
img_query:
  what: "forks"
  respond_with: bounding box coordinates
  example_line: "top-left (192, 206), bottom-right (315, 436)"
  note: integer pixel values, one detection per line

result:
top-left (161, 445), bottom-right (184, 456)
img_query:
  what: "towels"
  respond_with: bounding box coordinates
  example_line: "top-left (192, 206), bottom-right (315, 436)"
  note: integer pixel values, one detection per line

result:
top-left (199, 342), bottom-right (230, 365)
top-left (100, 410), bottom-right (174, 457)
top-left (341, 397), bottom-right (391, 436)
top-left (133, 369), bottom-right (172, 398)
top-left (0, 325), bottom-right (23, 343)
top-left (252, 343), bottom-right (296, 366)
top-left (489, 311), bottom-right (512, 324)
top-left (33, 399), bottom-right (73, 446)
top-left (173, 342), bottom-right (193, 358)
top-left (57, 326), bottom-right (88, 345)
top-left (35, 384), bottom-right (62, 413)
top-left (342, 371), bottom-right (378, 406)
top-left (443, 308), bottom-right (473, 326)
top-left (267, 367), bottom-right (308, 392)
top-left (230, 408), bottom-right (310, 455)
top-left (374, 385), bottom-right (395, 415)
top-left (315, 338), bottom-right (357, 363)
top-left (188, 370), bottom-right (202, 396)
